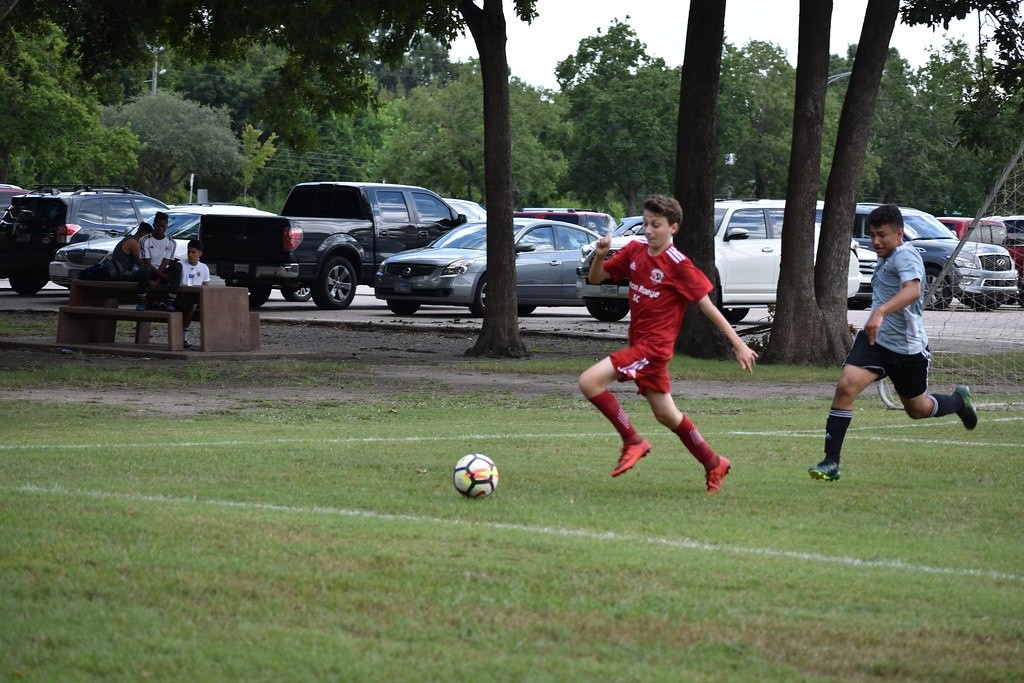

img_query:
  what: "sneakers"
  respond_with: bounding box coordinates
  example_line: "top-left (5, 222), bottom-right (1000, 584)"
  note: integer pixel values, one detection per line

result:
top-left (164, 294), bottom-right (177, 311)
top-left (611, 439), bottom-right (652, 477)
top-left (954, 384), bottom-right (978, 431)
top-left (807, 459), bottom-right (841, 482)
top-left (703, 456), bottom-right (731, 496)
top-left (183, 339), bottom-right (193, 348)
top-left (135, 292), bottom-right (147, 311)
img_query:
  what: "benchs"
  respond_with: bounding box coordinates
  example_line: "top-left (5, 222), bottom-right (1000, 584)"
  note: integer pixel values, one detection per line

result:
top-left (57, 306), bottom-right (184, 351)
top-left (135, 309), bottom-right (261, 351)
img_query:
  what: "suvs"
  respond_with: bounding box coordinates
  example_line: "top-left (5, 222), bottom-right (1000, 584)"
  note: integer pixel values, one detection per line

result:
top-left (854, 202), bottom-right (1024, 314)
top-left (0, 185), bottom-right (171, 297)
top-left (514, 205), bottom-right (618, 239)
top-left (0, 183), bottom-right (33, 281)
top-left (572, 197), bottom-right (863, 323)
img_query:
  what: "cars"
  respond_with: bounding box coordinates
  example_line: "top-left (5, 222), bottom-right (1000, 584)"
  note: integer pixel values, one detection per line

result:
top-left (843, 250), bottom-right (882, 312)
top-left (372, 216), bottom-right (603, 320)
top-left (47, 200), bottom-right (280, 306)
top-left (443, 197), bottom-right (488, 223)
top-left (608, 214), bottom-right (646, 239)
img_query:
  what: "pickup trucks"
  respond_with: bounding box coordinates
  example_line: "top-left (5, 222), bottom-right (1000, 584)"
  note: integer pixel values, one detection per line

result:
top-left (196, 181), bottom-right (468, 310)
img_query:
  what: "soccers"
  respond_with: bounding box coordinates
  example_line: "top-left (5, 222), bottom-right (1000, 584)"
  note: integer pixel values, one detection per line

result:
top-left (453, 453), bottom-right (499, 500)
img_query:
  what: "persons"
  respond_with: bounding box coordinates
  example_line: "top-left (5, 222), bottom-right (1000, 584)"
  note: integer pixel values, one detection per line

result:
top-left (111, 212), bottom-right (211, 347)
top-left (579, 195), bottom-right (758, 494)
top-left (808, 205), bottom-right (978, 480)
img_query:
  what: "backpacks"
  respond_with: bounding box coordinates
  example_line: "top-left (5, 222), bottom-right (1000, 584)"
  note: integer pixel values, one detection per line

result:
top-left (77, 261), bottom-right (117, 280)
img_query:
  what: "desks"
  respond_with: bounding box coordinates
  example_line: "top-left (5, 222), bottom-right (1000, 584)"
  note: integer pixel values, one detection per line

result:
top-left (69, 279), bottom-right (250, 353)
